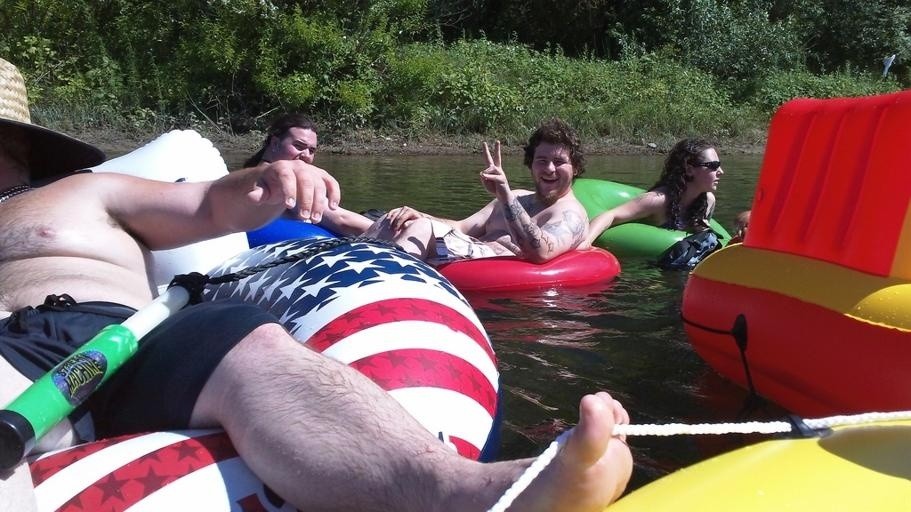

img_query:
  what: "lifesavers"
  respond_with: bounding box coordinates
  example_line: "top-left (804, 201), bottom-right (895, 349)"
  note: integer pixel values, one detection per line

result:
top-left (427, 246), bottom-right (620, 297)
top-left (572, 178), bottom-right (733, 262)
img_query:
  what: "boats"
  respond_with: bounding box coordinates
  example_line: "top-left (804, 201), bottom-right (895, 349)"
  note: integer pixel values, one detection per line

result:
top-left (673, 91), bottom-right (911, 420)
top-left (1, 128), bottom-right (499, 510)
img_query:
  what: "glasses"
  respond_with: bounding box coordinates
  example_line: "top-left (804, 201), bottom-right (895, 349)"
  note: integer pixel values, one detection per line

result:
top-left (695, 161), bottom-right (721, 171)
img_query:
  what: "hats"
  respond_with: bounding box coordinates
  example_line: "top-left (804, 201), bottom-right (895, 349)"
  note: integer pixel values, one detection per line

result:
top-left (0, 57), bottom-right (106, 170)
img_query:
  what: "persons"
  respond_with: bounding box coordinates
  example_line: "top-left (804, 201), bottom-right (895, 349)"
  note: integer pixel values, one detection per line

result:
top-left (578, 137), bottom-right (725, 252)
top-left (1, 55), bottom-right (635, 512)
top-left (355, 118), bottom-right (590, 265)
top-left (244, 114), bottom-right (380, 237)
top-left (735, 210), bottom-right (752, 242)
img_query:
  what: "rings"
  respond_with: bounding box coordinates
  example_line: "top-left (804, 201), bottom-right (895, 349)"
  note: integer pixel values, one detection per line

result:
top-left (742, 225), bottom-right (749, 230)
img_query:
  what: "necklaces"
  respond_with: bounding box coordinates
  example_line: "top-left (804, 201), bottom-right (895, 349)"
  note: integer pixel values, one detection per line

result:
top-left (259, 158), bottom-right (272, 165)
